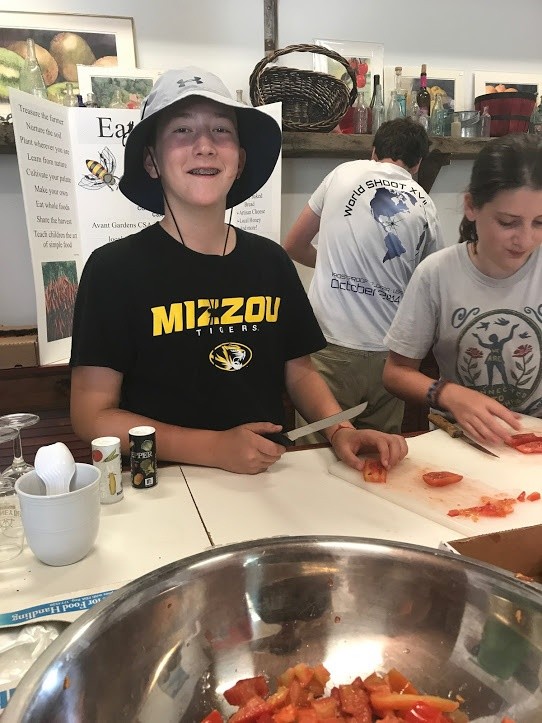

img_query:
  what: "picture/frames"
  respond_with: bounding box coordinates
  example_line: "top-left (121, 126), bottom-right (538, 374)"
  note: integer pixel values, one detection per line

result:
top-left (311, 36), bottom-right (384, 131)
top-left (0, 11), bottom-right (137, 121)
top-left (473, 69), bottom-right (542, 108)
top-left (389, 66), bottom-right (462, 137)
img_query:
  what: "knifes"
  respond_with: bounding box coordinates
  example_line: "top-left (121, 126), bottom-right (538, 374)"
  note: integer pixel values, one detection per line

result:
top-left (427, 413), bottom-right (502, 459)
top-left (264, 401), bottom-right (369, 448)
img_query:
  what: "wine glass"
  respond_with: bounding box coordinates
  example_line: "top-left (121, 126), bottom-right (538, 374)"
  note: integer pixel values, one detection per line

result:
top-left (0, 413), bottom-right (40, 562)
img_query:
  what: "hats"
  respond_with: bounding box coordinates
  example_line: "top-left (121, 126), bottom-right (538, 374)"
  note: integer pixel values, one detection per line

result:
top-left (120, 67), bottom-right (281, 215)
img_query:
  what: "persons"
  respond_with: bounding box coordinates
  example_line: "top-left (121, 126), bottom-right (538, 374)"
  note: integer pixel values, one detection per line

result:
top-left (284, 115), bottom-right (440, 446)
top-left (71, 66), bottom-right (409, 475)
top-left (381, 133), bottom-right (542, 442)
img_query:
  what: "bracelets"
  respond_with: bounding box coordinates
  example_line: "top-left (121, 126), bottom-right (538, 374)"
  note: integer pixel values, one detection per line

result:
top-left (434, 380), bottom-right (452, 412)
top-left (426, 381), bottom-right (439, 411)
top-left (331, 424), bottom-right (353, 441)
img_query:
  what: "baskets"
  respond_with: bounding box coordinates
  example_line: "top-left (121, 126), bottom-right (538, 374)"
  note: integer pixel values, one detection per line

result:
top-left (250, 44), bottom-right (358, 132)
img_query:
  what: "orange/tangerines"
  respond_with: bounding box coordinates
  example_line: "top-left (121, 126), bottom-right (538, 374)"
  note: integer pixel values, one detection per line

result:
top-left (486, 84), bottom-right (518, 93)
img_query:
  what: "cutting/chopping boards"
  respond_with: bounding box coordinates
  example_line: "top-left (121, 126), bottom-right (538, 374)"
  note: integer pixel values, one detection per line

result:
top-left (328, 413), bottom-right (542, 539)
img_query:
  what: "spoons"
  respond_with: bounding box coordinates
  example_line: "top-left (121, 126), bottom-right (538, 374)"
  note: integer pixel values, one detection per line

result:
top-left (34, 442), bottom-right (77, 496)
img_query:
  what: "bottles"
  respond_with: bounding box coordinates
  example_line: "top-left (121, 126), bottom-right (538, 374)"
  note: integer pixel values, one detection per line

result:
top-left (355, 63), bottom-right (446, 138)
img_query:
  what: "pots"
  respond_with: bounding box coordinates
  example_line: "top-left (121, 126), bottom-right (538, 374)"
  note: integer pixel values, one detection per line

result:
top-left (448, 110), bottom-right (492, 139)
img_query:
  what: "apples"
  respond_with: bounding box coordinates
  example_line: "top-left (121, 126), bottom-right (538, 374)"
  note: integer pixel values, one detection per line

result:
top-left (348, 57), bottom-right (368, 87)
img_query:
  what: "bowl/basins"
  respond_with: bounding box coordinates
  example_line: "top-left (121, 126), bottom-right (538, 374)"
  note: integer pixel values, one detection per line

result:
top-left (1, 535), bottom-right (542, 723)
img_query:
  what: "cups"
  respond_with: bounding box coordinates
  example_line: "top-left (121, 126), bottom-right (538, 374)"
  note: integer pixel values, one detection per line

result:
top-left (128, 426), bottom-right (157, 489)
top-left (91, 436), bottom-right (123, 505)
top-left (15, 463), bottom-right (101, 566)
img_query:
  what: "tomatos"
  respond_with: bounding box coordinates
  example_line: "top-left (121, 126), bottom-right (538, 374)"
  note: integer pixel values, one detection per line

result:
top-left (201, 663), bottom-right (517, 723)
top-left (505, 433), bottom-right (542, 453)
top-left (363, 457), bottom-right (387, 481)
top-left (423, 472), bottom-right (464, 486)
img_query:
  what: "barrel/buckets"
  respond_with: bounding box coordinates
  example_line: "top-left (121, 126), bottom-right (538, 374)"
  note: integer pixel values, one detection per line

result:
top-left (474, 93), bottom-right (536, 137)
